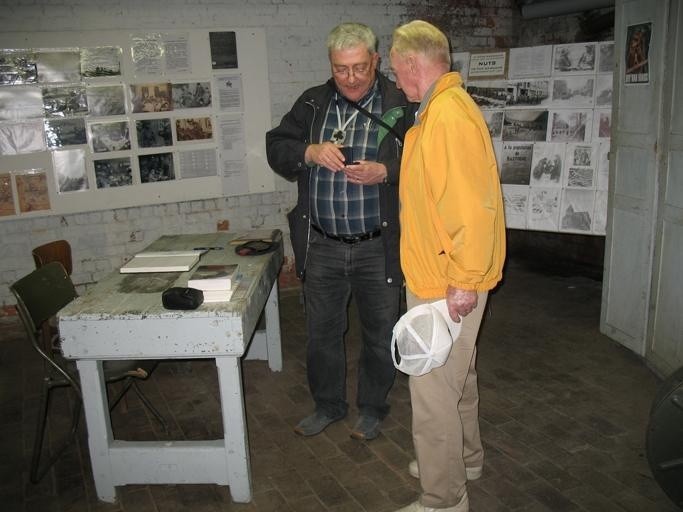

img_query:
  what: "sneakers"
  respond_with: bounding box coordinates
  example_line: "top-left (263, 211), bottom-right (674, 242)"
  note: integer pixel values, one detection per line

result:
top-left (350, 415), bottom-right (381, 440)
top-left (409, 459), bottom-right (483, 480)
top-left (394, 490), bottom-right (469, 512)
top-left (294, 409), bottom-right (346, 436)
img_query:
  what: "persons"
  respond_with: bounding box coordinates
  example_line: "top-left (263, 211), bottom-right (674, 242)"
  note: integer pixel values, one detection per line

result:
top-left (385, 19), bottom-right (506, 511)
top-left (264, 21), bottom-right (420, 442)
top-left (130, 81), bottom-right (211, 148)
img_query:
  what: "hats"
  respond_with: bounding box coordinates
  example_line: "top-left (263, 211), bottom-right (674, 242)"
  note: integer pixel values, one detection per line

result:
top-left (391, 299), bottom-right (463, 377)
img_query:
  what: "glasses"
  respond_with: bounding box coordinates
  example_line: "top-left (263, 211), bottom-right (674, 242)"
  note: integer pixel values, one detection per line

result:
top-left (334, 58), bottom-right (373, 78)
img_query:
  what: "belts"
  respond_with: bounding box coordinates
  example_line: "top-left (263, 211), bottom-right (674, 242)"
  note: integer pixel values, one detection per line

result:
top-left (311, 224), bottom-right (382, 244)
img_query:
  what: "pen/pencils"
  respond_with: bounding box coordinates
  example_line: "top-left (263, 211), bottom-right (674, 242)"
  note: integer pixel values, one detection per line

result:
top-left (193, 247), bottom-right (224, 250)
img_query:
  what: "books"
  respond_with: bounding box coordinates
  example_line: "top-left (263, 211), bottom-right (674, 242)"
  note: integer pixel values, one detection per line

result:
top-left (119, 248), bottom-right (210, 273)
top-left (187, 263), bottom-right (241, 302)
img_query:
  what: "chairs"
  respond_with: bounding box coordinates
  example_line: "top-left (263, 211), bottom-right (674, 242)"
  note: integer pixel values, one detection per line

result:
top-left (9, 260), bottom-right (167, 482)
top-left (30, 239), bottom-right (73, 276)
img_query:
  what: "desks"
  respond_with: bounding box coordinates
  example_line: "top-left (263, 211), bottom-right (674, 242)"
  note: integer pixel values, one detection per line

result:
top-left (54, 230), bottom-right (288, 501)
